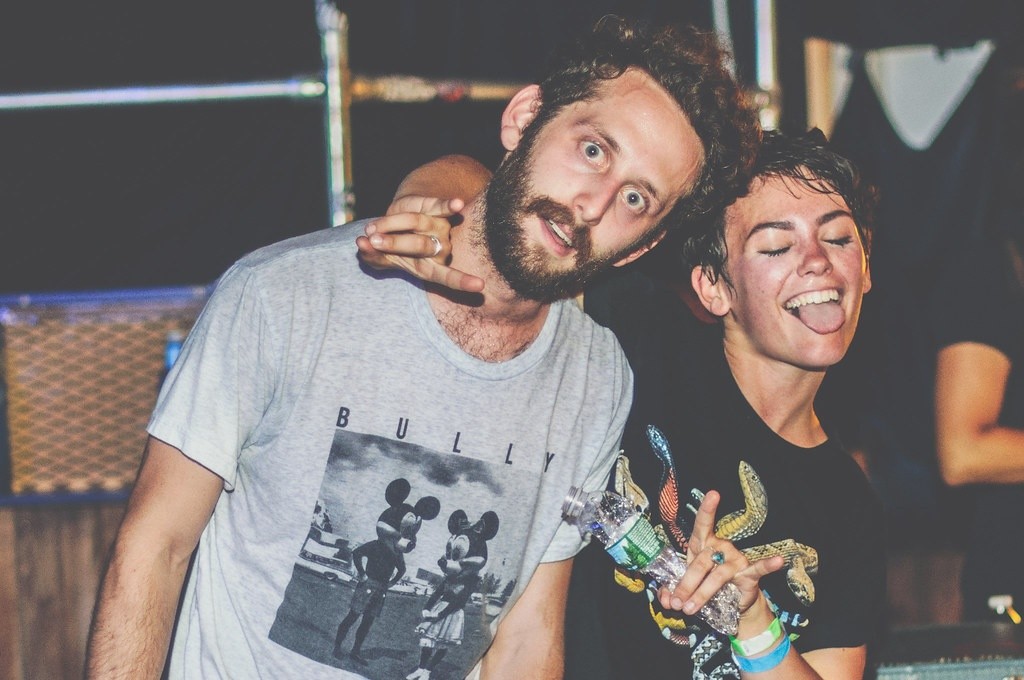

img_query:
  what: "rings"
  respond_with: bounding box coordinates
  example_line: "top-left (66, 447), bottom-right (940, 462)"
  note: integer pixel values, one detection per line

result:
top-left (710, 545), bottom-right (724, 566)
top-left (430, 235), bottom-right (442, 257)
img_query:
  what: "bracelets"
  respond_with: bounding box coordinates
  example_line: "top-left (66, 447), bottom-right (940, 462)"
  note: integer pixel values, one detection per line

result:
top-left (728, 616), bottom-right (791, 673)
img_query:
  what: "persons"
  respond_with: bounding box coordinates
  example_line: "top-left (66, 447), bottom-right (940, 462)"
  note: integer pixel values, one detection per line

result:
top-left (356, 126), bottom-right (881, 680)
top-left (933, 187), bottom-right (1024, 624)
top-left (82, 12), bottom-right (764, 679)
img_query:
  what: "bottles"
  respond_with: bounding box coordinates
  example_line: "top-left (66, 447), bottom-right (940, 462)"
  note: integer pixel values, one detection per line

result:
top-left (560, 486), bottom-right (742, 636)
